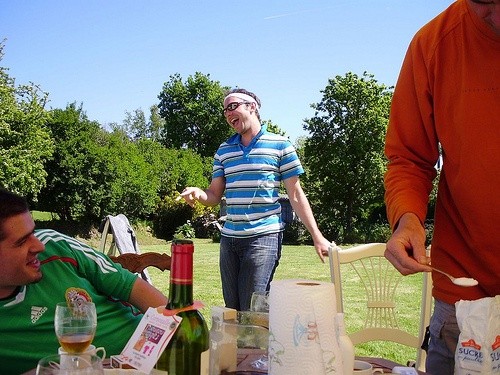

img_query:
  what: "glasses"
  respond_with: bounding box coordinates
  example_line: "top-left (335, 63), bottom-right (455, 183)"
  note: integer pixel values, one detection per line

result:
top-left (222, 101), bottom-right (250, 116)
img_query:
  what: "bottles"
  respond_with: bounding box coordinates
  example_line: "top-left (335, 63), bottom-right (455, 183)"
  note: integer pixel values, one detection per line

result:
top-left (155, 240), bottom-right (210, 375)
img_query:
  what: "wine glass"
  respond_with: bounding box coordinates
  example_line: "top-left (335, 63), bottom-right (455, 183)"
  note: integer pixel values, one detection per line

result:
top-left (53, 301), bottom-right (97, 369)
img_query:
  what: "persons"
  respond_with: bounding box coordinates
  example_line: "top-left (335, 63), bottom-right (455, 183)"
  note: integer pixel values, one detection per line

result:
top-left (181, 89), bottom-right (342, 347)
top-left (384, 0), bottom-right (500, 375)
top-left (0, 187), bottom-right (169, 375)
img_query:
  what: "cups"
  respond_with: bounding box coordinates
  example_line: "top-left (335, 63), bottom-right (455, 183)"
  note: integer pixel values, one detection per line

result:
top-left (57, 344), bottom-right (106, 370)
top-left (352, 359), bottom-right (384, 375)
top-left (35, 353), bottom-right (104, 375)
top-left (208, 311), bottom-right (269, 375)
top-left (250, 290), bottom-right (269, 350)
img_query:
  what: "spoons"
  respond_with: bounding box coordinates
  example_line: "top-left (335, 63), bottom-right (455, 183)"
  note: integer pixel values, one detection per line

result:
top-left (426, 265), bottom-right (479, 287)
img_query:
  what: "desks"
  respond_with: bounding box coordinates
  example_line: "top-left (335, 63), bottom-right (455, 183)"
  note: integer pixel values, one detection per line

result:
top-left (26, 348), bottom-right (431, 375)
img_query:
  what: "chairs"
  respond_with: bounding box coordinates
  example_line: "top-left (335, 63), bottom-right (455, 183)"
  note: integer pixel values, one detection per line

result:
top-left (330, 241), bottom-right (432, 371)
top-left (109, 252), bottom-right (171, 273)
